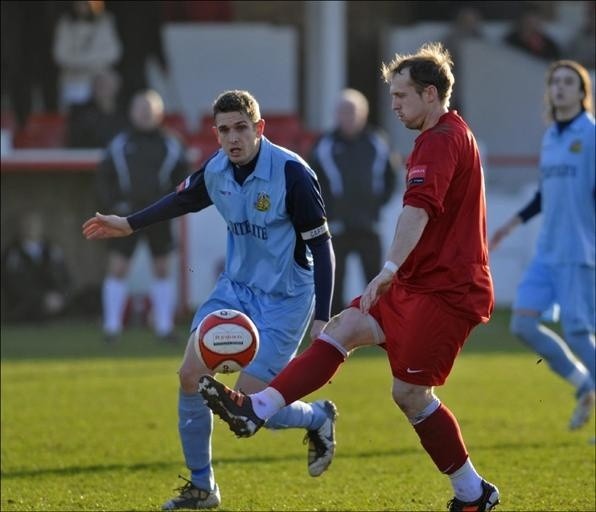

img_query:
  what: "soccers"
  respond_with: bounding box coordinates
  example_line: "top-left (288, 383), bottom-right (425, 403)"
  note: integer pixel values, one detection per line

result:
top-left (193, 308), bottom-right (260, 374)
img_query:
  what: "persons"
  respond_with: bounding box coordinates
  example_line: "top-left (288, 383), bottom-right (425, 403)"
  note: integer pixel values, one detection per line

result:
top-left (97, 87), bottom-right (188, 347)
top-left (309, 87), bottom-right (398, 320)
top-left (53, 1), bottom-right (123, 145)
top-left (198, 56), bottom-right (500, 512)
top-left (82, 92), bottom-right (338, 512)
top-left (489, 60), bottom-right (596, 430)
top-left (5, 210), bottom-right (77, 320)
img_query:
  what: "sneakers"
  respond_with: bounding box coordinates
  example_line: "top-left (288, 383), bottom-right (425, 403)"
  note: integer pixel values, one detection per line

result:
top-left (160, 474), bottom-right (222, 512)
top-left (301, 398), bottom-right (339, 478)
top-left (567, 379), bottom-right (594, 431)
top-left (446, 478), bottom-right (501, 512)
top-left (196, 373), bottom-right (265, 440)
